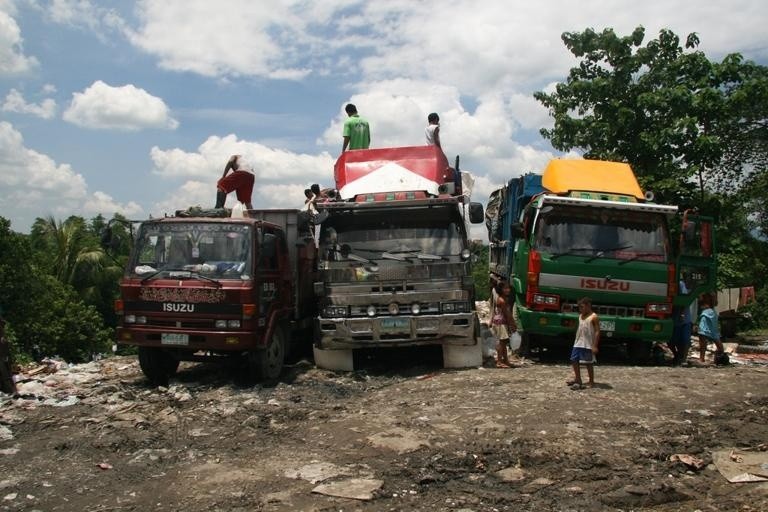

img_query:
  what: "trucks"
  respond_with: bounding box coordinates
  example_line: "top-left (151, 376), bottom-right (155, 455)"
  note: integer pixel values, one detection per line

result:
top-left (295, 142), bottom-right (489, 373)
top-left (486, 157), bottom-right (720, 366)
top-left (97, 207), bottom-right (318, 391)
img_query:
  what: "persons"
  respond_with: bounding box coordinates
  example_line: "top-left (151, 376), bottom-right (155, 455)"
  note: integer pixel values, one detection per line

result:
top-left (305, 189), bottom-right (314, 204)
top-left (342, 104), bottom-right (370, 152)
top-left (565, 297), bottom-right (600, 388)
top-left (491, 281), bottom-right (518, 368)
top-left (311, 184), bottom-right (328, 204)
top-left (215, 155), bottom-right (255, 208)
top-left (667, 281), bottom-right (692, 364)
top-left (425, 112), bottom-right (440, 146)
top-left (697, 290), bottom-right (723, 362)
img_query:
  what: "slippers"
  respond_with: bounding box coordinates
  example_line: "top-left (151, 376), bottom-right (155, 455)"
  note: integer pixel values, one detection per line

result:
top-left (566, 380), bottom-right (586, 390)
top-left (495, 361), bottom-right (515, 368)
